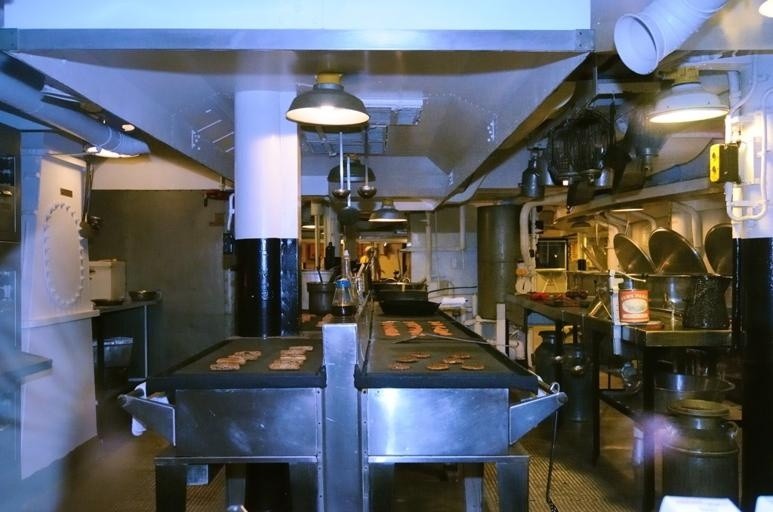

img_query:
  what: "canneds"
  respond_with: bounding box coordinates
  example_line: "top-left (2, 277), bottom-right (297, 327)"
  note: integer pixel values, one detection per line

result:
top-left (620, 289), bottom-right (650, 323)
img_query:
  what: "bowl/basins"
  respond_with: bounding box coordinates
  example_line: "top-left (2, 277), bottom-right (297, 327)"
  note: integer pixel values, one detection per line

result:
top-left (636, 368), bottom-right (734, 412)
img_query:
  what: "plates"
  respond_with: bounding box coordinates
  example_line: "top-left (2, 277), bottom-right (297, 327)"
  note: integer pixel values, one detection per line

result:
top-left (90, 298), bottom-right (123, 305)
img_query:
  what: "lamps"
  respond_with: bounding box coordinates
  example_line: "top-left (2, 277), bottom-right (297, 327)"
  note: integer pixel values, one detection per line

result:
top-left (647, 75), bottom-right (728, 128)
top-left (284, 69), bottom-right (373, 130)
top-left (325, 153), bottom-right (376, 187)
top-left (369, 200), bottom-right (408, 225)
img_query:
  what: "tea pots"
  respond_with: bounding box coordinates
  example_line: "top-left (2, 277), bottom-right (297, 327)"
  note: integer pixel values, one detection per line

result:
top-left (329, 274), bottom-right (358, 317)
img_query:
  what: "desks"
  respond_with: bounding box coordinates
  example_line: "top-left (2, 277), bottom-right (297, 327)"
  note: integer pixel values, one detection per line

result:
top-left (92, 294), bottom-right (159, 410)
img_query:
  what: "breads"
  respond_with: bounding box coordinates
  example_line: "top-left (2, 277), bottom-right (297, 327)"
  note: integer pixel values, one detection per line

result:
top-left (210, 351), bottom-right (261, 370)
top-left (268, 345), bottom-right (313, 369)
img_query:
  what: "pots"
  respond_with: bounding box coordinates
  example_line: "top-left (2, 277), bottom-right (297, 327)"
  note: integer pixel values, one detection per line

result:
top-left (377, 299), bottom-right (440, 315)
top-left (128, 287), bottom-right (156, 302)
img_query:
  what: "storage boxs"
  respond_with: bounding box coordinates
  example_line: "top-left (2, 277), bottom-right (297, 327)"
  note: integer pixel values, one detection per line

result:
top-left (90, 261), bottom-right (126, 302)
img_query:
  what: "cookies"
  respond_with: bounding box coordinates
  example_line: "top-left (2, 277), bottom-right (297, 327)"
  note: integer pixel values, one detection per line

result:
top-left (388, 350), bottom-right (486, 372)
